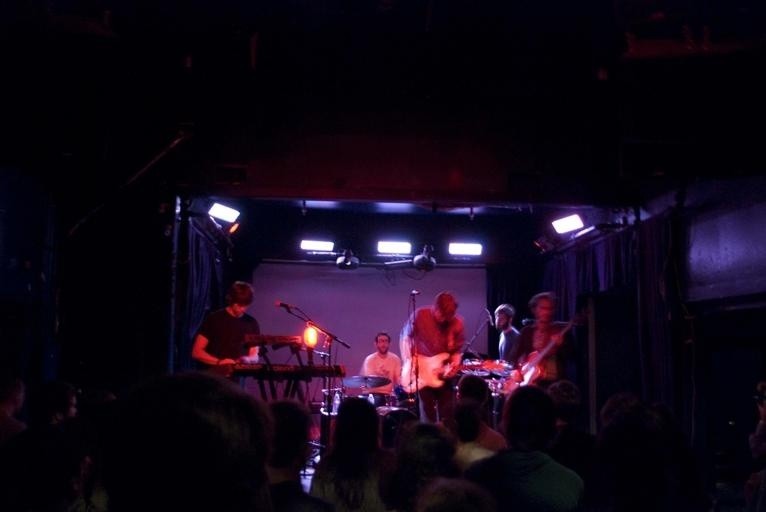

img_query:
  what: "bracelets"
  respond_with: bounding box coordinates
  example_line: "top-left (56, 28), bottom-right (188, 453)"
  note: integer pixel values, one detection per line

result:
top-left (214, 357), bottom-right (222, 366)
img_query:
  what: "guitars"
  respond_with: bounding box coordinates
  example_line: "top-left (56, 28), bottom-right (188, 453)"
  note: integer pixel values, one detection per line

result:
top-left (498, 309), bottom-right (592, 398)
top-left (399, 352), bottom-right (515, 394)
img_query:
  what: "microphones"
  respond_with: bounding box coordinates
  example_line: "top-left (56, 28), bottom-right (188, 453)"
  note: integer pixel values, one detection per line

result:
top-left (522, 319), bottom-right (535, 325)
top-left (274, 299), bottom-right (292, 308)
top-left (487, 310), bottom-right (494, 327)
top-left (410, 289), bottom-right (420, 295)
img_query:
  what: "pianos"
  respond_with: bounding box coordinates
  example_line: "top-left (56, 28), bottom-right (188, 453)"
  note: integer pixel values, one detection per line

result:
top-left (222, 363), bottom-right (347, 378)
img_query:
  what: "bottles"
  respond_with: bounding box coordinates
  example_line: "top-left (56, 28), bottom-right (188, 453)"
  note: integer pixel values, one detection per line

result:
top-left (332, 393), bottom-right (341, 414)
top-left (367, 393), bottom-right (375, 405)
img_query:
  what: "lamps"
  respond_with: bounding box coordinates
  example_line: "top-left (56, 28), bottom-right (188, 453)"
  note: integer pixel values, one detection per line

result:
top-left (206, 201), bottom-right (592, 259)
top-left (335, 247), bottom-right (361, 270)
top-left (411, 200), bottom-right (439, 272)
top-left (303, 327), bottom-right (317, 364)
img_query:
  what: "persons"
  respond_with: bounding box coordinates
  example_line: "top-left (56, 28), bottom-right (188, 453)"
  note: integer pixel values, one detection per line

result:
top-left (511, 291), bottom-right (567, 387)
top-left (1, 359), bottom-right (766, 511)
top-left (494, 304), bottom-right (522, 367)
top-left (399, 291), bottom-right (467, 436)
top-left (191, 281), bottom-right (262, 368)
top-left (357, 331), bottom-right (404, 397)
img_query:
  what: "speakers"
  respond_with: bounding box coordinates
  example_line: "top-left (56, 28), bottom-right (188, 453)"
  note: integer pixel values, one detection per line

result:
top-left (320, 406), bottom-right (338, 459)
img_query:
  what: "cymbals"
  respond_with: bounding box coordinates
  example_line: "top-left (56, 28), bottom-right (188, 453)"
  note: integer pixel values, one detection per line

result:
top-left (339, 375), bottom-right (392, 388)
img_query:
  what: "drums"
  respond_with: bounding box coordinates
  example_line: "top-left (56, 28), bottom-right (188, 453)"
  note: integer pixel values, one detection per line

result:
top-left (487, 359), bottom-right (514, 377)
top-left (462, 358), bottom-right (489, 378)
